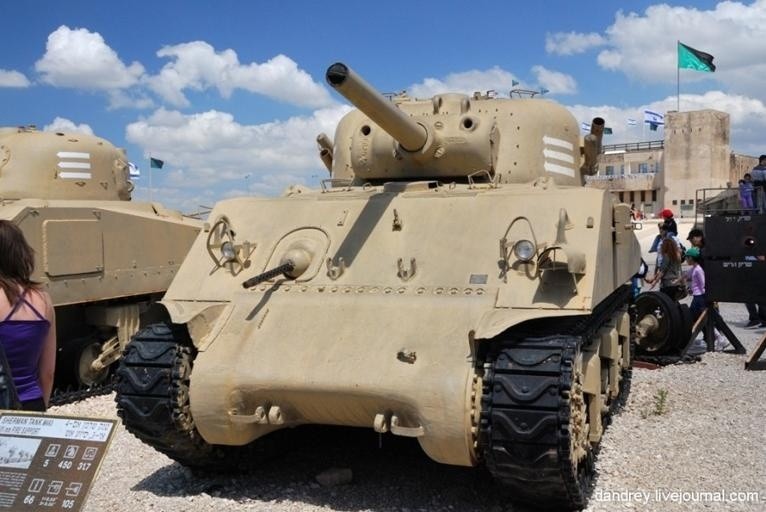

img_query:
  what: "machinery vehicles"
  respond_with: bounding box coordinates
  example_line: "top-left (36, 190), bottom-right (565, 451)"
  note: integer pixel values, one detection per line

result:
top-left (0, 61), bottom-right (692, 506)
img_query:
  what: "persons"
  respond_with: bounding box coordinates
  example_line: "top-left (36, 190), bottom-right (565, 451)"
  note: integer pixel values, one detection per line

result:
top-left (630, 155), bottom-right (766, 352)
top-left (0, 220), bottom-right (56, 411)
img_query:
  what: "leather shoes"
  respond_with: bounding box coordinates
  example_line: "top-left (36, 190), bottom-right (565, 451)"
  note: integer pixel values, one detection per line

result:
top-left (747, 320), bottom-right (766, 328)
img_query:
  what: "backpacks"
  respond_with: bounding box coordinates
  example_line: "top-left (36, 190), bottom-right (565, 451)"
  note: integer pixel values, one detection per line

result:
top-left (679, 242), bottom-right (686, 263)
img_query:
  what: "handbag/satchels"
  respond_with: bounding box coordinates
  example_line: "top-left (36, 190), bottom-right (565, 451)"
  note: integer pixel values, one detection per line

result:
top-left (662, 269), bottom-right (682, 288)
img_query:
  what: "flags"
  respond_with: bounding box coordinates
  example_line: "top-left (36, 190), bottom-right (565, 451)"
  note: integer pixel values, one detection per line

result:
top-left (128, 162), bottom-right (140, 179)
top-left (644, 110), bottom-right (665, 125)
top-left (151, 158), bottom-right (163, 169)
top-left (512, 80), bottom-right (519, 87)
top-left (604, 128), bottom-right (612, 134)
top-left (582, 123), bottom-right (590, 131)
top-left (541, 88), bottom-right (550, 95)
top-left (679, 42), bottom-right (715, 72)
top-left (628, 119), bottom-right (636, 126)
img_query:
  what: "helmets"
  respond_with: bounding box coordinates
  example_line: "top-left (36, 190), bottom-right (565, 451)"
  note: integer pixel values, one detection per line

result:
top-left (682, 246), bottom-right (700, 258)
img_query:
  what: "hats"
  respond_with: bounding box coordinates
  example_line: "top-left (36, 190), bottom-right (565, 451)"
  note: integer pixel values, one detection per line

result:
top-left (660, 209), bottom-right (673, 218)
top-left (687, 229), bottom-right (703, 240)
